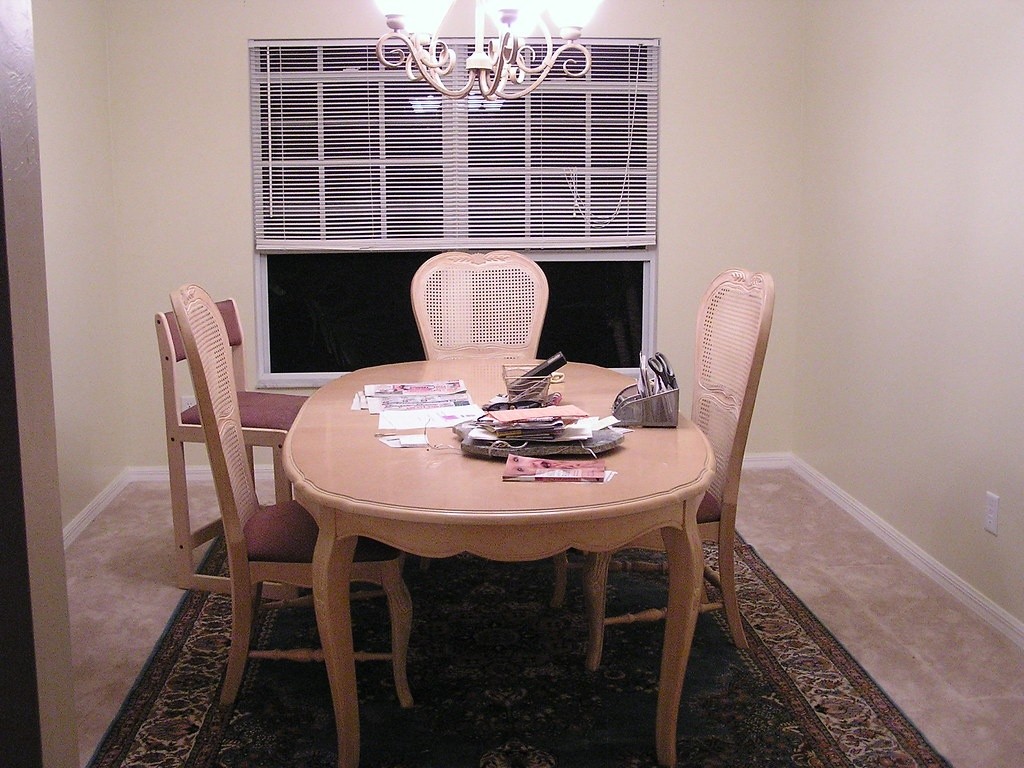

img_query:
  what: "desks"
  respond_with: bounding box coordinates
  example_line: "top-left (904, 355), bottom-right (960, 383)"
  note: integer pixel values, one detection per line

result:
top-left (281, 358), bottom-right (717, 768)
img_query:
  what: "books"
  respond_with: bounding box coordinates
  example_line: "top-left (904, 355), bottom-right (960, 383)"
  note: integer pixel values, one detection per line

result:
top-left (502, 453), bottom-right (606, 482)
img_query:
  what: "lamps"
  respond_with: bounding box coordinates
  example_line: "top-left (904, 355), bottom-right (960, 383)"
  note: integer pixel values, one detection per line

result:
top-left (375, 0), bottom-right (603, 100)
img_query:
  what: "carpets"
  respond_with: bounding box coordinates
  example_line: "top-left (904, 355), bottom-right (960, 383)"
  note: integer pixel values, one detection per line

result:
top-left (85, 517), bottom-right (953, 768)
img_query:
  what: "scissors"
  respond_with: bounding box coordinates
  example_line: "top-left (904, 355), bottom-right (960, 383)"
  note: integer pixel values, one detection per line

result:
top-left (648, 352), bottom-right (675, 417)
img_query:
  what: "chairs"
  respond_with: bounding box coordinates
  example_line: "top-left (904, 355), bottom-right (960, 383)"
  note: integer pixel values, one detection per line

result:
top-left (411, 250), bottom-right (549, 362)
top-left (172, 283), bottom-right (418, 708)
top-left (158, 298), bottom-right (322, 602)
top-left (548, 269), bottom-right (777, 668)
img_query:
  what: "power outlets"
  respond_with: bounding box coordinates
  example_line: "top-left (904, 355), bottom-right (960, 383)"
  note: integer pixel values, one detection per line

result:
top-left (983, 491), bottom-right (999, 537)
